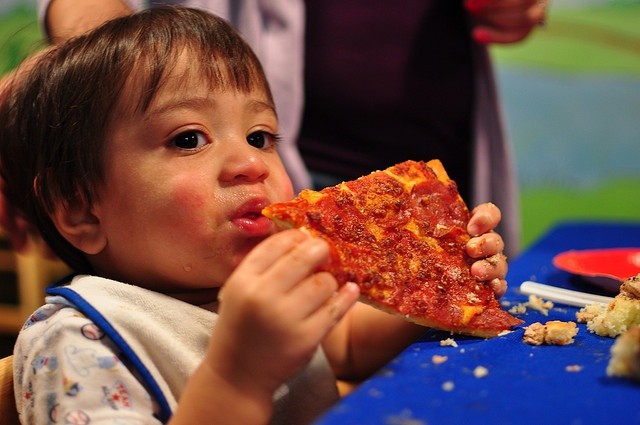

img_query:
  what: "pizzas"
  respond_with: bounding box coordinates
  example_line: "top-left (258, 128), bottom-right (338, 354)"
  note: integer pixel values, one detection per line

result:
top-left (261, 158), bottom-right (526, 338)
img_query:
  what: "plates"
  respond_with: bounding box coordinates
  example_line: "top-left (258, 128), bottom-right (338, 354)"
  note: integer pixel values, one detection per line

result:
top-left (553, 247), bottom-right (640, 288)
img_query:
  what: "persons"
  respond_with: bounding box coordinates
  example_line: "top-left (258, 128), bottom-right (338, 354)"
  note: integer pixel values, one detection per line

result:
top-left (1, 4), bottom-right (509, 425)
top-left (39, 0), bottom-right (549, 264)
